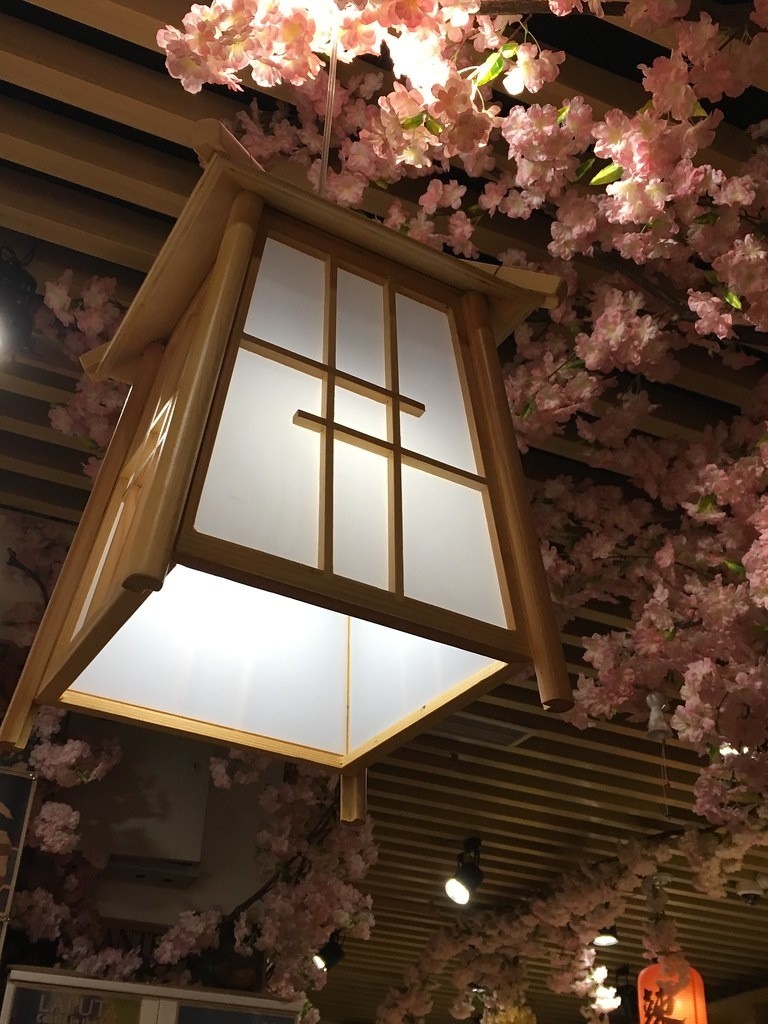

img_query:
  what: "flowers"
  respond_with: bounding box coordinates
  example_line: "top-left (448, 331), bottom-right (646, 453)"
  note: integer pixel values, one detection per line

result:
top-left (0, 0), bottom-right (768, 1024)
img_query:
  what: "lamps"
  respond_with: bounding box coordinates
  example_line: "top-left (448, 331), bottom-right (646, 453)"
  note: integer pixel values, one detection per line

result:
top-left (312, 929), bottom-right (350, 976)
top-left (588, 914), bottom-right (618, 949)
top-left (604, 965), bottom-right (638, 1024)
top-left (0, 40), bottom-right (575, 831)
top-left (445, 837), bottom-right (485, 906)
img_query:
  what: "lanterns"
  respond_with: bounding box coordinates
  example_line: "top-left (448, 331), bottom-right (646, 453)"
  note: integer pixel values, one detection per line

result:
top-left (0, 150), bottom-right (574, 826)
top-left (637, 941), bottom-right (706, 1024)
